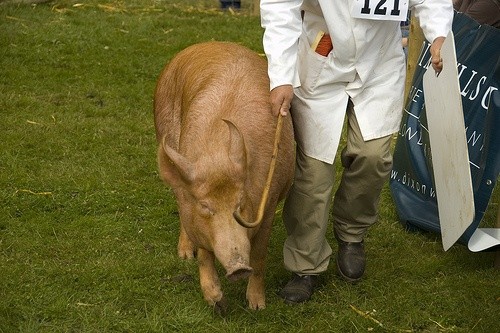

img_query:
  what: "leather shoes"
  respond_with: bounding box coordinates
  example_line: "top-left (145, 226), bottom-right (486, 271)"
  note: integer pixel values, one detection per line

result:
top-left (276, 271), bottom-right (315, 306)
top-left (333, 226), bottom-right (367, 282)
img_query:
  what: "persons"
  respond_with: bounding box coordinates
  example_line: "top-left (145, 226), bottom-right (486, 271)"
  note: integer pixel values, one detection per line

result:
top-left (258, 0), bottom-right (455, 305)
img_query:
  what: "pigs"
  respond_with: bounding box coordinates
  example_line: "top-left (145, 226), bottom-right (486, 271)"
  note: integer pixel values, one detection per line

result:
top-left (153, 40), bottom-right (296, 312)
